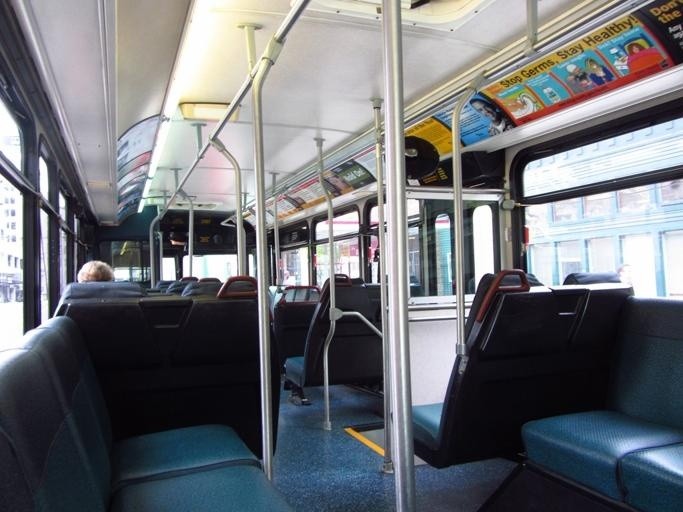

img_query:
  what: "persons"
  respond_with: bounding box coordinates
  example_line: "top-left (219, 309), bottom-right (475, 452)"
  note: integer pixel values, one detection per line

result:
top-left (627, 43), bottom-right (645, 53)
top-left (470, 98), bottom-right (514, 136)
top-left (566, 57), bottom-right (614, 90)
top-left (77, 260), bottom-right (115, 281)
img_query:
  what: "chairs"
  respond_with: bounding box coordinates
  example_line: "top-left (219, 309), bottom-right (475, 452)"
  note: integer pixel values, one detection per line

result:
top-left (49, 268), bottom-right (420, 454)
top-left (0, 313), bottom-right (295, 512)
top-left (410, 266), bottom-right (683, 511)
